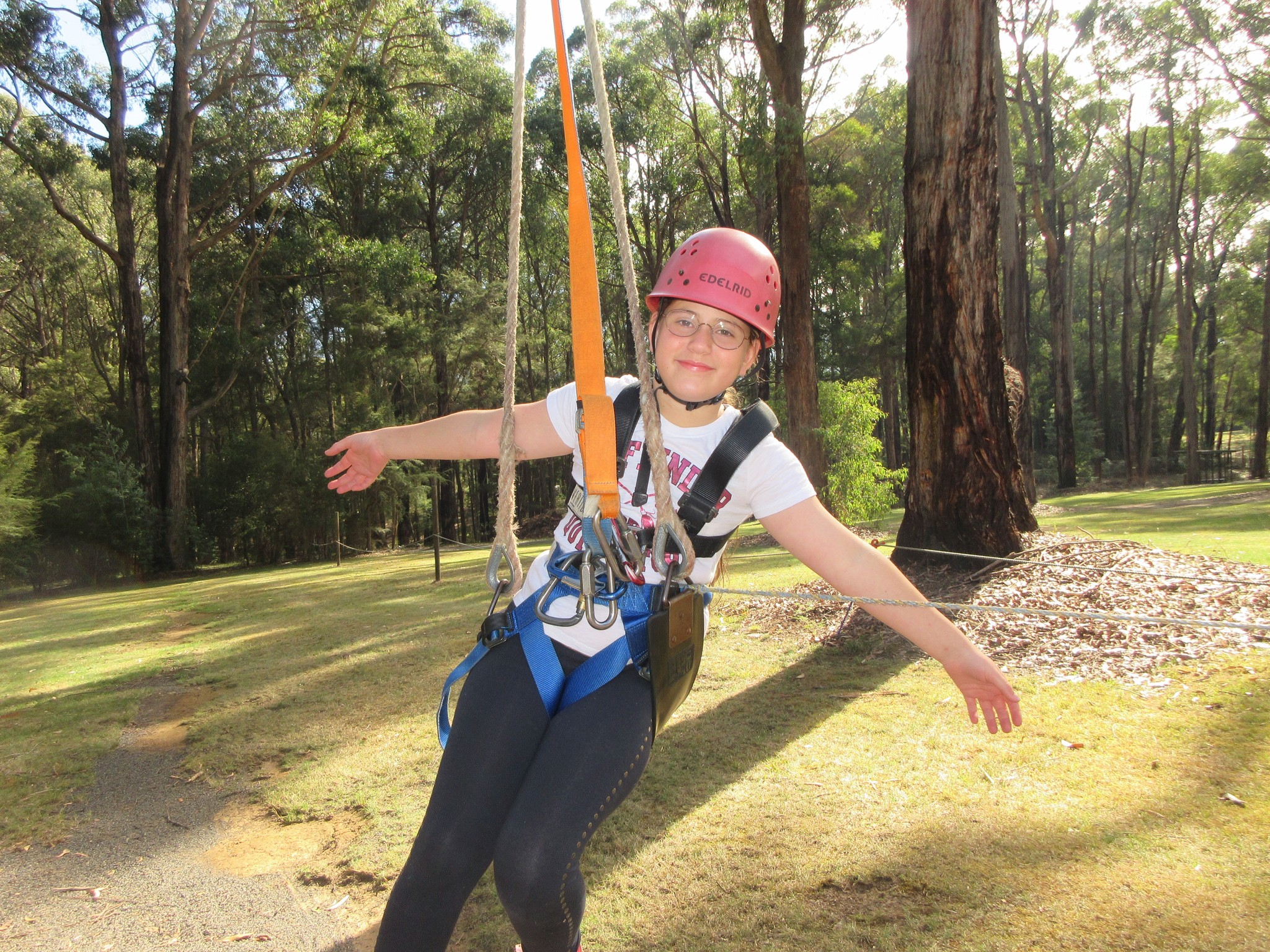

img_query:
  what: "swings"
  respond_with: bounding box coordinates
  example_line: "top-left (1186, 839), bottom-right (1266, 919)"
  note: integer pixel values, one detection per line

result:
top-left (486, 0), bottom-right (725, 742)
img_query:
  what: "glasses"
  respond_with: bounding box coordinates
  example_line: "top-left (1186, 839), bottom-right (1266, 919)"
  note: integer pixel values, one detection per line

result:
top-left (661, 309), bottom-right (752, 350)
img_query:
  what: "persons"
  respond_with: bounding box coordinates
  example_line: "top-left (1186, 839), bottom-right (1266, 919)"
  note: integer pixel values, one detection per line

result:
top-left (324, 227), bottom-right (1021, 952)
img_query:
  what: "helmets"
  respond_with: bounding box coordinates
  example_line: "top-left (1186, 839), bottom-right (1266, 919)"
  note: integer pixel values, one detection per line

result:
top-left (644, 227), bottom-right (782, 350)
